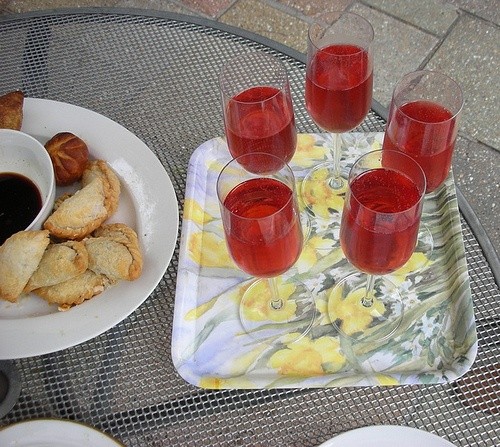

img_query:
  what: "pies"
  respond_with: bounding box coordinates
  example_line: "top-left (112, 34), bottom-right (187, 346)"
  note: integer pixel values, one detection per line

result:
top-left (0, 89), bottom-right (24, 132)
top-left (0, 158), bottom-right (143, 307)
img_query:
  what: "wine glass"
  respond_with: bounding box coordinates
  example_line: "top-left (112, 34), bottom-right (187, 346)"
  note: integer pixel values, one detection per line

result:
top-left (215, 151), bottom-right (317, 347)
top-left (300, 10), bottom-right (376, 220)
top-left (379, 69), bottom-right (466, 276)
top-left (327, 148), bottom-right (427, 343)
top-left (218, 51), bottom-right (313, 248)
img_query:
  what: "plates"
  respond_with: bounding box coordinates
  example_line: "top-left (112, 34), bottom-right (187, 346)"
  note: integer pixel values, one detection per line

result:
top-left (0, 418), bottom-right (126, 447)
top-left (0, 97), bottom-right (179, 361)
top-left (318, 424), bottom-right (458, 447)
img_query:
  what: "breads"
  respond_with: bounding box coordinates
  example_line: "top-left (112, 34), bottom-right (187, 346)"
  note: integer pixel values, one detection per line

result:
top-left (45, 131), bottom-right (89, 187)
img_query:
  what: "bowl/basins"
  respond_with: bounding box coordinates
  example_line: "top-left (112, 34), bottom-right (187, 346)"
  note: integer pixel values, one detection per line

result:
top-left (0, 127), bottom-right (57, 248)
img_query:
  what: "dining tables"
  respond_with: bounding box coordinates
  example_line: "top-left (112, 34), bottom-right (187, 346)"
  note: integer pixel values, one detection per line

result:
top-left (0, 11), bottom-right (500, 447)
